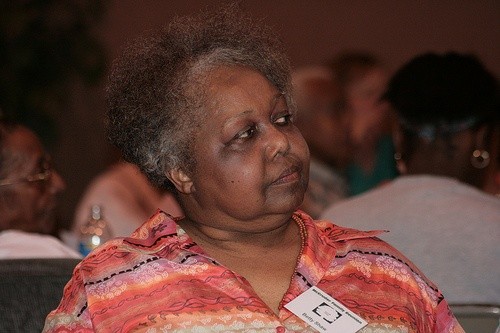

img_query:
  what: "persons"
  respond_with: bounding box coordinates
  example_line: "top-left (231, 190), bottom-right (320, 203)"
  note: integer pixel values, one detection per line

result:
top-left (0, 48), bottom-right (500, 305)
top-left (44, 0), bottom-right (466, 333)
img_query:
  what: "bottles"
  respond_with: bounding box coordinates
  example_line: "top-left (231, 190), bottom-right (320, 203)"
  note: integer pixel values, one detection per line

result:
top-left (79, 203), bottom-right (111, 256)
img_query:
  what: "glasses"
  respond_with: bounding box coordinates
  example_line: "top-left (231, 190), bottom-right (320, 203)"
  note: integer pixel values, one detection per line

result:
top-left (0, 154), bottom-right (52, 186)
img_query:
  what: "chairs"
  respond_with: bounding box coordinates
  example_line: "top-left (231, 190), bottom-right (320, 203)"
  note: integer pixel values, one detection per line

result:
top-left (0, 258), bottom-right (82, 333)
top-left (447, 305), bottom-right (500, 333)
